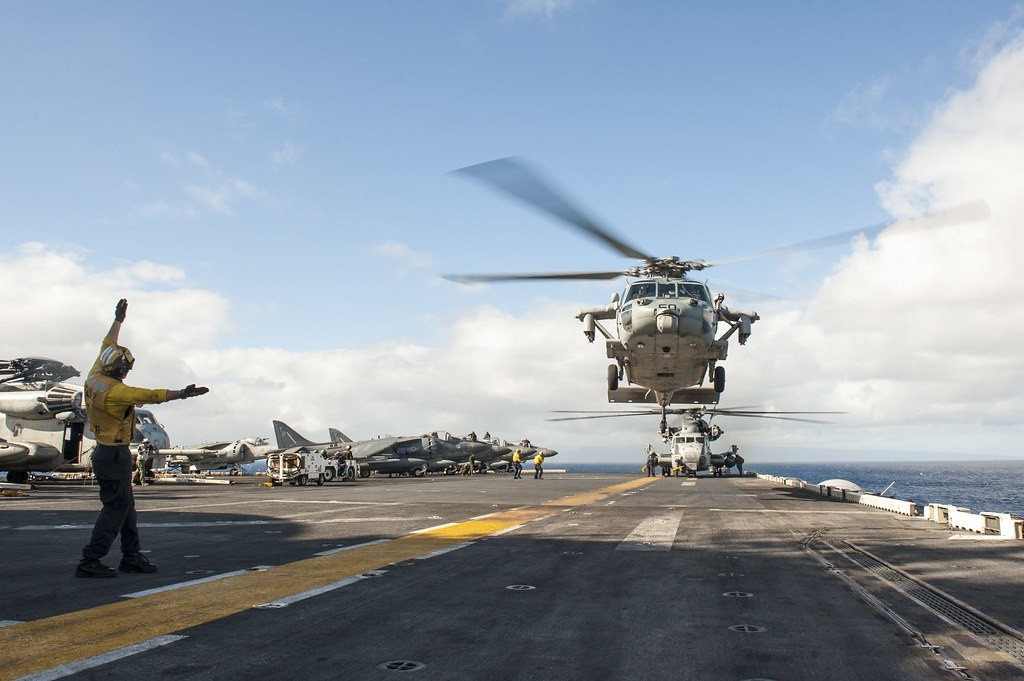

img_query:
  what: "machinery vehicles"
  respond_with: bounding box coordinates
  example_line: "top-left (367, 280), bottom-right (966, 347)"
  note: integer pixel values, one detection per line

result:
top-left (323, 459), bottom-right (361, 482)
top-left (266, 450), bottom-right (326, 487)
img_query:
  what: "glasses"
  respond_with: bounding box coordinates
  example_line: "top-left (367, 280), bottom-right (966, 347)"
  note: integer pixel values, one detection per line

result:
top-left (145, 442), bottom-right (149, 443)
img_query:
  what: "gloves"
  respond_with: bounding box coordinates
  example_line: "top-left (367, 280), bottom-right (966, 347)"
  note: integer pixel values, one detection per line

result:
top-left (181, 384), bottom-right (208, 399)
top-left (144, 445), bottom-right (150, 450)
top-left (115, 298), bottom-right (128, 322)
top-left (149, 445), bottom-right (153, 450)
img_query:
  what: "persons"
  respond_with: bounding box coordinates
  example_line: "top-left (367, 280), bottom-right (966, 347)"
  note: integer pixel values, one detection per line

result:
top-left (674, 459), bottom-right (689, 479)
top-left (468, 432), bottom-right (477, 441)
top-left (469, 454), bottom-right (475, 477)
top-left (534, 451), bottom-right (544, 480)
top-left (634, 285), bottom-right (648, 298)
top-left (525, 439), bottom-right (530, 443)
top-left (486, 432), bottom-right (490, 437)
top-left (132, 438), bottom-right (150, 487)
top-left (343, 445), bottom-right (353, 460)
top-left (459, 464), bottom-right (468, 476)
top-left (75, 298), bottom-right (209, 578)
top-left (512, 448), bottom-right (525, 479)
top-left (690, 286), bottom-right (700, 295)
top-left (647, 455), bottom-right (656, 477)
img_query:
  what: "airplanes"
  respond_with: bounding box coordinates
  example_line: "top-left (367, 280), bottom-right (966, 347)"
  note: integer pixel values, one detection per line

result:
top-left (264, 420), bottom-right (558, 476)
top-left (0, 356), bottom-right (170, 486)
top-left (150, 436), bottom-right (277, 475)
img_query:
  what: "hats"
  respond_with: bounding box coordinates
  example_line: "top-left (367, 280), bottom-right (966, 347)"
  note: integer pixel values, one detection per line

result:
top-left (516, 449), bottom-right (520, 452)
top-left (142, 438), bottom-right (150, 442)
top-left (539, 451), bottom-right (543, 455)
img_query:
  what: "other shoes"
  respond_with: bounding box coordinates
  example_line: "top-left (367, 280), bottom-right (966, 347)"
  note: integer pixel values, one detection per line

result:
top-left (514, 477), bottom-right (517, 479)
top-left (539, 478), bottom-right (543, 479)
top-left (535, 477), bottom-right (537, 479)
top-left (652, 474), bottom-right (655, 476)
top-left (518, 476), bottom-right (522, 479)
top-left (648, 475), bottom-right (650, 477)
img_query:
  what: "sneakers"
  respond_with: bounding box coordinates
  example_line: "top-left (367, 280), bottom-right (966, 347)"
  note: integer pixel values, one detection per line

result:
top-left (75, 561), bottom-right (118, 577)
top-left (119, 552), bottom-right (157, 573)
top-left (131, 482), bottom-right (136, 487)
top-left (142, 483), bottom-right (149, 486)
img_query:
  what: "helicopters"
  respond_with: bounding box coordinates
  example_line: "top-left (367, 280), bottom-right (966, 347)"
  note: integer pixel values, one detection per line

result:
top-left (438, 159), bottom-right (985, 433)
top-left (545, 407), bottom-right (852, 476)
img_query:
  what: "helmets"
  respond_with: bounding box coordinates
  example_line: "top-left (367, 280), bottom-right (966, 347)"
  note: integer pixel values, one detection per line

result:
top-left (99, 343), bottom-right (135, 371)
